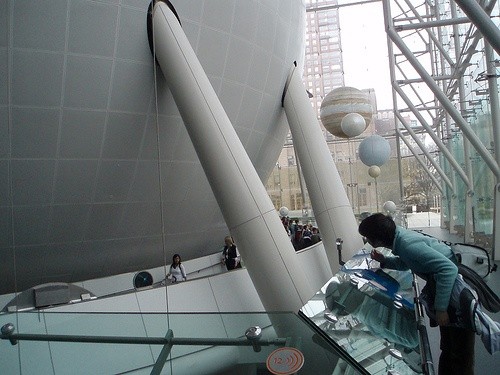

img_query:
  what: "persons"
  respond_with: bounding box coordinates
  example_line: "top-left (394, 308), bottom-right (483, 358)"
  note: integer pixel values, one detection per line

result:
top-left (222, 235), bottom-right (242, 271)
top-left (166, 253), bottom-right (186, 284)
top-left (357, 211), bottom-right (500, 375)
top-left (279, 217), bottom-right (322, 253)
